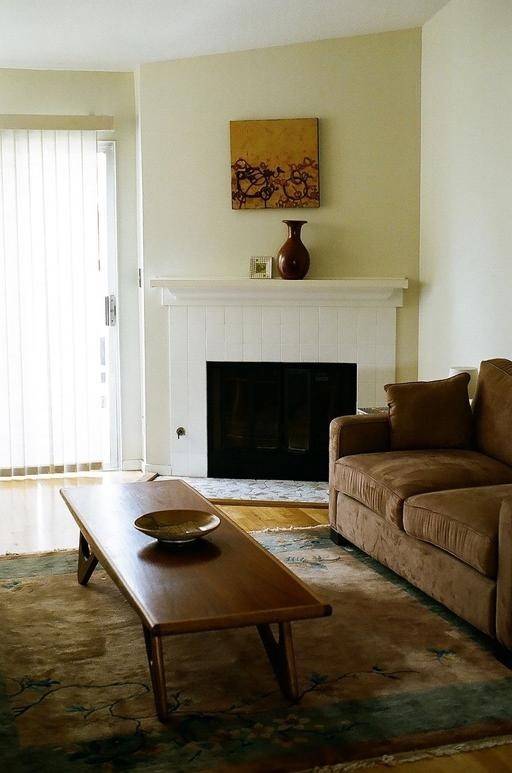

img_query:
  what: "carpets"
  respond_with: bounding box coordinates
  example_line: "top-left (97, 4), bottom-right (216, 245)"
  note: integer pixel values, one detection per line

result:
top-left (0, 522), bottom-right (511, 771)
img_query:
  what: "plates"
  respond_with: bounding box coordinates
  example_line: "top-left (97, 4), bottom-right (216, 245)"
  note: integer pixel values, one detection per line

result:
top-left (133, 509), bottom-right (222, 545)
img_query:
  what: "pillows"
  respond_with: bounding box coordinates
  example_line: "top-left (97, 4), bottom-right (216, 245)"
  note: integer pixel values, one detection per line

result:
top-left (382, 373), bottom-right (477, 450)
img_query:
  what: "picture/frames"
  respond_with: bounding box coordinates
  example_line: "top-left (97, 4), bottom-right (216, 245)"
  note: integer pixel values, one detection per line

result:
top-left (250, 256), bottom-right (273, 279)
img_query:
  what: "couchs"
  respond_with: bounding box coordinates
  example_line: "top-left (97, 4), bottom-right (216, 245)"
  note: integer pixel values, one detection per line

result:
top-left (325, 358), bottom-right (512, 654)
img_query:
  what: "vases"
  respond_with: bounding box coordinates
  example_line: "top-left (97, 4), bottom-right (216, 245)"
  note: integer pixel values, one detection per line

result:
top-left (278, 219), bottom-right (311, 279)
